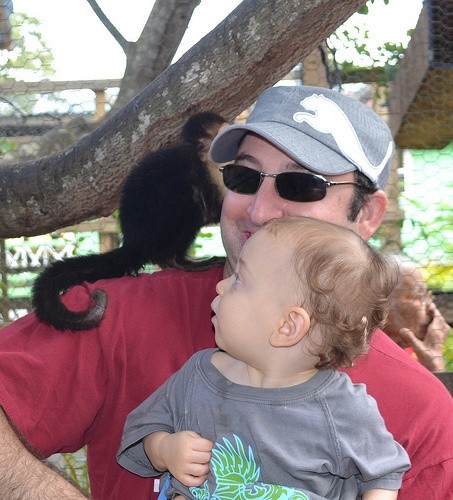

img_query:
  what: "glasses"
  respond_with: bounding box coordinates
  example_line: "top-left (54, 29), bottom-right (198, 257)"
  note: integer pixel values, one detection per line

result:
top-left (219, 164), bottom-right (362, 202)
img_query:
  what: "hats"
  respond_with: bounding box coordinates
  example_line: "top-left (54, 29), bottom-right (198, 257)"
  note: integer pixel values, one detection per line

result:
top-left (209, 86), bottom-right (395, 190)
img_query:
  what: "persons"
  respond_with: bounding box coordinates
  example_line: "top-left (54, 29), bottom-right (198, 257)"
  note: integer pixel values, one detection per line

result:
top-left (0, 85), bottom-right (452, 500)
top-left (114, 213), bottom-right (411, 500)
top-left (375, 253), bottom-right (451, 396)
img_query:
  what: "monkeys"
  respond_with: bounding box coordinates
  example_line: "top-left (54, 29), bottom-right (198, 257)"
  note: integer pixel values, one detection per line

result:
top-left (32, 111), bottom-right (236, 330)
